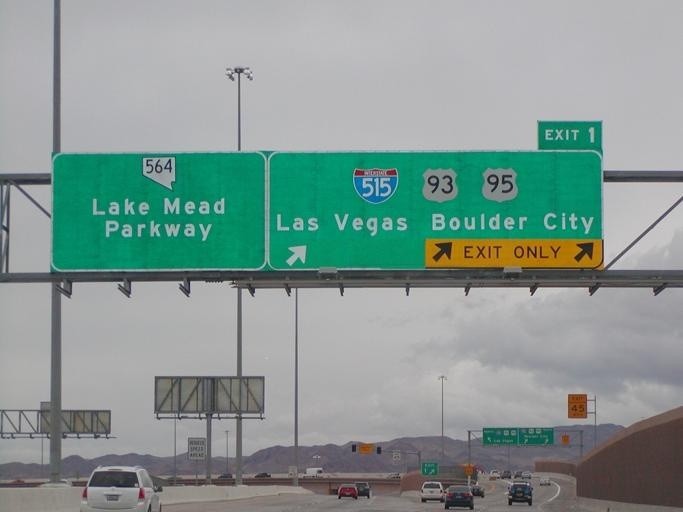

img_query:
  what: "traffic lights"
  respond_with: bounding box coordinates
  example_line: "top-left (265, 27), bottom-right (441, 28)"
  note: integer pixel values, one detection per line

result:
top-left (376, 447), bottom-right (381, 455)
top-left (351, 445), bottom-right (355, 452)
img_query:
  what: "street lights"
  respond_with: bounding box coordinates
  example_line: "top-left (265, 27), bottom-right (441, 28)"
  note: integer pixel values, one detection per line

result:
top-left (224, 431), bottom-right (229, 475)
top-left (222, 66), bottom-right (253, 490)
top-left (437, 374), bottom-right (446, 455)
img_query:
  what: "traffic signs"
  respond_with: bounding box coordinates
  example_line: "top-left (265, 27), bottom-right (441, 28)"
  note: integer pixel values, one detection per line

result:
top-left (358, 444), bottom-right (373, 454)
top-left (265, 150), bottom-right (605, 274)
top-left (567, 393), bottom-right (587, 419)
top-left (483, 427), bottom-right (518, 447)
top-left (537, 119), bottom-right (602, 150)
top-left (420, 463), bottom-right (438, 477)
top-left (517, 428), bottom-right (552, 446)
top-left (51, 152), bottom-right (265, 272)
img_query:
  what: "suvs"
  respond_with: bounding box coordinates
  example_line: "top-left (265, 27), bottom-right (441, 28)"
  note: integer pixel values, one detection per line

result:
top-left (500, 471), bottom-right (510, 479)
top-left (79, 465), bottom-right (163, 512)
top-left (355, 482), bottom-right (371, 499)
top-left (506, 480), bottom-right (533, 507)
top-left (489, 470), bottom-right (500, 479)
top-left (512, 469), bottom-right (522, 479)
top-left (521, 471), bottom-right (531, 479)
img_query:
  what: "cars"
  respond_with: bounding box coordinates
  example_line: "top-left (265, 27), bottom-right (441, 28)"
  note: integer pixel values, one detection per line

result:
top-left (218, 474), bottom-right (232, 479)
top-left (538, 476), bottom-right (550, 486)
top-left (470, 485), bottom-right (484, 498)
top-left (443, 485), bottom-right (474, 510)
top-left (337, 484), bottom-right (358, 500)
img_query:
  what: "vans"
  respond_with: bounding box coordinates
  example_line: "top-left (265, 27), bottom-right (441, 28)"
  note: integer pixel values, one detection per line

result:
top-left (255, 472), bottom-right (271, 478)
top-left (420, 481), bottom-right (444, 504)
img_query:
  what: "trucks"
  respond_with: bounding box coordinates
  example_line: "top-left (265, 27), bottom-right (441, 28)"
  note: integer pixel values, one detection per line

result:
top-left (306, 468), bottom-right (324, 478)
